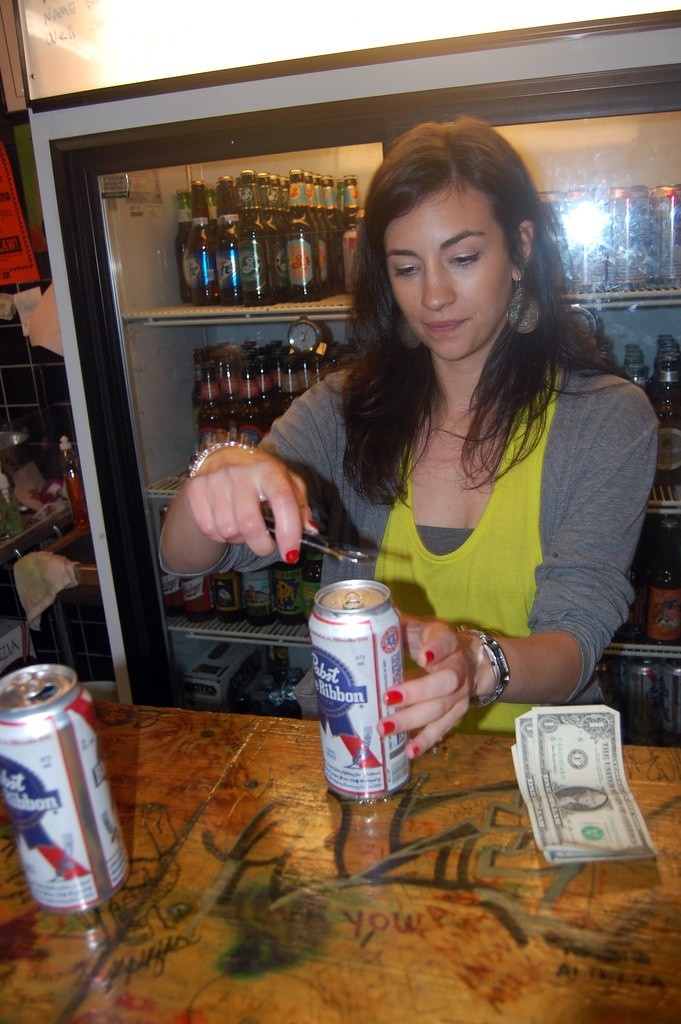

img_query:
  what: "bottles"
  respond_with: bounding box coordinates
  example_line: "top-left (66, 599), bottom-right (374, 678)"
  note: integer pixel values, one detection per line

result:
top-left (188, 337), bottom-right (363, 475)
top-left (59, 434), bottom-right (91, 532)
top-left (156, 498), bottom-right (328, 624)
top-left (233, 646), bottom-right (301, 720)
top-left (593, 334), bottom-right (681, 486)
top-left (606, 514), bottom-right (680, 648)
top-left (174, 167), bottom-right (363, 307)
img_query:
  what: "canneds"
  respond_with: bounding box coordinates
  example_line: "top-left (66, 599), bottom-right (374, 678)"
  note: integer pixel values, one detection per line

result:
top-left (623, 656), bottom-right (681, 737)
top-left (0, 663), bottom-right (129, 912)
top-left (309, 580), bottom-right (411, 801)
top-left (536, 183), bottom-right (680, 287)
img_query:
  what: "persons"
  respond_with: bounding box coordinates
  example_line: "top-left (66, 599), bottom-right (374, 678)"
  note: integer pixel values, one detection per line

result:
top-left (157, 117), bottom-right (661, 760)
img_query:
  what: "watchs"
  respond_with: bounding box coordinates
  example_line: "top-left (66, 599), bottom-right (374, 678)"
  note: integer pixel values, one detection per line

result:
top-left (458, 624), bottom-right (509, 708)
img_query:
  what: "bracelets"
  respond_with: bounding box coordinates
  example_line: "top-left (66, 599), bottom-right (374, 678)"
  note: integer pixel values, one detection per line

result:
top-left (189, 439), bottom-right (257, 475)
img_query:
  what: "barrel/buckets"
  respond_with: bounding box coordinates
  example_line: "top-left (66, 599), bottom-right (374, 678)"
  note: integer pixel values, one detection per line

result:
top-left (0, 431), bottom-right (31, 509)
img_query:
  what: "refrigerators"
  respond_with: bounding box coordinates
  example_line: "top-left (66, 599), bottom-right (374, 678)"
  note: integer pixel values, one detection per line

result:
top-left (13, 0), bottom-right (681, 751)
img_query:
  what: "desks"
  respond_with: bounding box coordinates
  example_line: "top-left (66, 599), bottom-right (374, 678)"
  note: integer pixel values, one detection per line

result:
top-left (0, 706), bottom-right (680, 1024)
